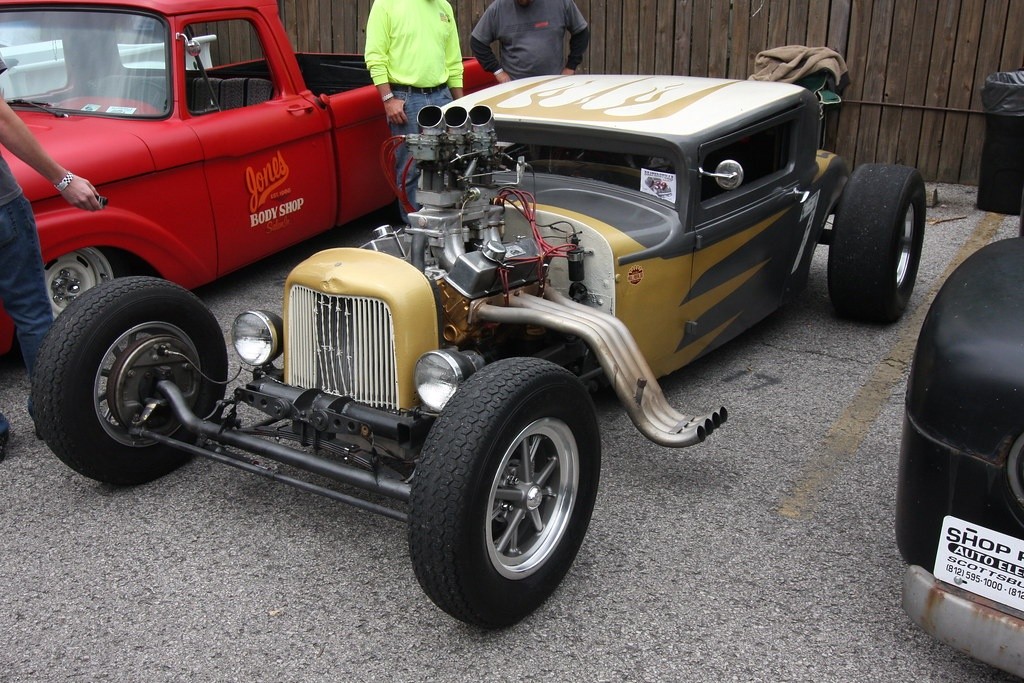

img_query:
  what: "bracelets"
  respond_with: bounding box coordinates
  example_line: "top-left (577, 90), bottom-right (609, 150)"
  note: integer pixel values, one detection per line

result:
top-left (54, 171), bottom-right (74, 192)
top-left (493, 68), bottom-right (503, 75)
top-left (382, 92), bottom-right (394, 102)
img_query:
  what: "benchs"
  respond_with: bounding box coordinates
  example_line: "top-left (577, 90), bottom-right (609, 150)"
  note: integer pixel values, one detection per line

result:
top-left (99, 75), bottom-right (271, 112)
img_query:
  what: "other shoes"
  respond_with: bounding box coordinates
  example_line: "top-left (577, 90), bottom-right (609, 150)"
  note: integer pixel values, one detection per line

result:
top-left (0, 426), bottom-right (8, 464)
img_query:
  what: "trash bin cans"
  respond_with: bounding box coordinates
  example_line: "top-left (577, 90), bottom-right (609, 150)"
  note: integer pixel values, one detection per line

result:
top-left (975, 71), bottom-right (1024, 215)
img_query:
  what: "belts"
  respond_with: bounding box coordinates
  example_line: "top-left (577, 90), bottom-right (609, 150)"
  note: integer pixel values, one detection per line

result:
top-left (389, 83), bottom-right (448, 95)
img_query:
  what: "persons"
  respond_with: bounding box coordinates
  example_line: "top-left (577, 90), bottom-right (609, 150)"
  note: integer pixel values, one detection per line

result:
top-left (365, 0), bottom-right (465, 223)
top-left (0, 56), bottom-right (104, 463)
top-left (470, 0), bottom-right (590, 84)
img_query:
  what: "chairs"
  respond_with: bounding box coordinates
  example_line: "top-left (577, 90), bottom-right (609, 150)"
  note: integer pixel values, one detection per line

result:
top-left (797, 67), bottom-right (841, 148)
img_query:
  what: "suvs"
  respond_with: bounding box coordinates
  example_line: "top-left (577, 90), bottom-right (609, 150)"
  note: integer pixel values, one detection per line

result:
top-left (22, 74), bottom-right (931, 633)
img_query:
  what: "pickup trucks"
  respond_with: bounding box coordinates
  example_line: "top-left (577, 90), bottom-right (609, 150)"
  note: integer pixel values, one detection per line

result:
top-left (1, 0), bottom-right (494, 352)
